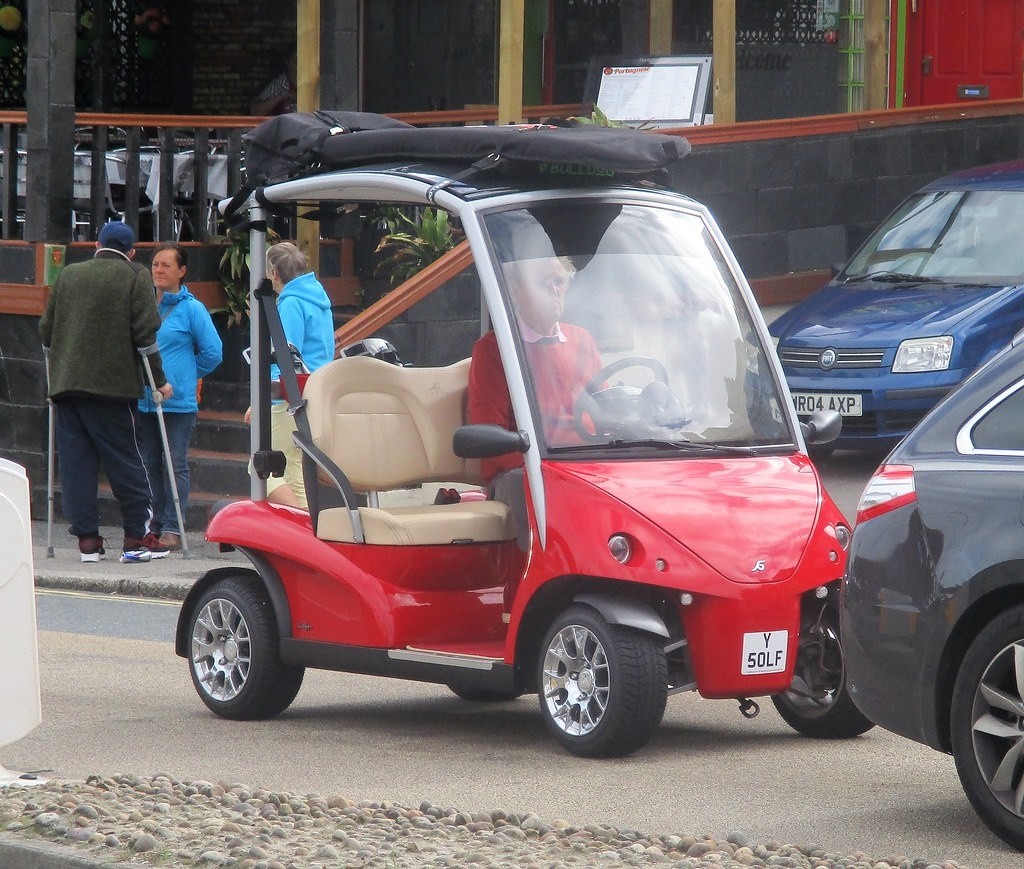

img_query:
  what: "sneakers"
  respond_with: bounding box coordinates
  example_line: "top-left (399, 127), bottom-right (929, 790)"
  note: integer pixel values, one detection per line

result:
top-left (122, 535), bottom-right (171, 559)
top-left (81, 537), bottom-right (105, 561)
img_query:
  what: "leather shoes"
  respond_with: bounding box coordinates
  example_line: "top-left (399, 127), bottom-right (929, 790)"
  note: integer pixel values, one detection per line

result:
top-left (151, 532), bottom-right (181, 552)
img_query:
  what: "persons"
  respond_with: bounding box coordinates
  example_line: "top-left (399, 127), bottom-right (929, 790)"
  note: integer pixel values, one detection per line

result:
top-left (464, 241), bottom-right (611, 555)
top-left (242, 240), bottom-right (336, 424)
top-left (247, 399), bottom-right (312, 515)
top-left (136, 240), bottom-right (224, 552)
top-left (38, 220), bottom-right (171, 562)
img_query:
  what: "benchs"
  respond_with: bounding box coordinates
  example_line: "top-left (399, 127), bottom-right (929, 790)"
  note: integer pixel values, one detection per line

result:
top-left (296, 357), bottom-right (517, 547)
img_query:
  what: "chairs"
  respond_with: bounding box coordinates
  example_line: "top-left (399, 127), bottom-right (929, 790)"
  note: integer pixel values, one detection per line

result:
top-left (0, 126), bottom-right (245, 243)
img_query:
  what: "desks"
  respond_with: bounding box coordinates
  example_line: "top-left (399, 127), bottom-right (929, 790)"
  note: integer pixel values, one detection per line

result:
top-left (0, 149), bottom-right (227, 242)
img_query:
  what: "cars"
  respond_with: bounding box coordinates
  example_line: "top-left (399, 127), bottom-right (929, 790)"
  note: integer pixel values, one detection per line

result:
top-left (745, 159), bottom-right (1024, 462)
top-left (838, 327), bottom-right (1024, 854)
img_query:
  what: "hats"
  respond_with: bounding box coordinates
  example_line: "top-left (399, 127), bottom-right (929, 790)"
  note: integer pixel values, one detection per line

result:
top-left (98, 221), bottom-right (133, 251)
